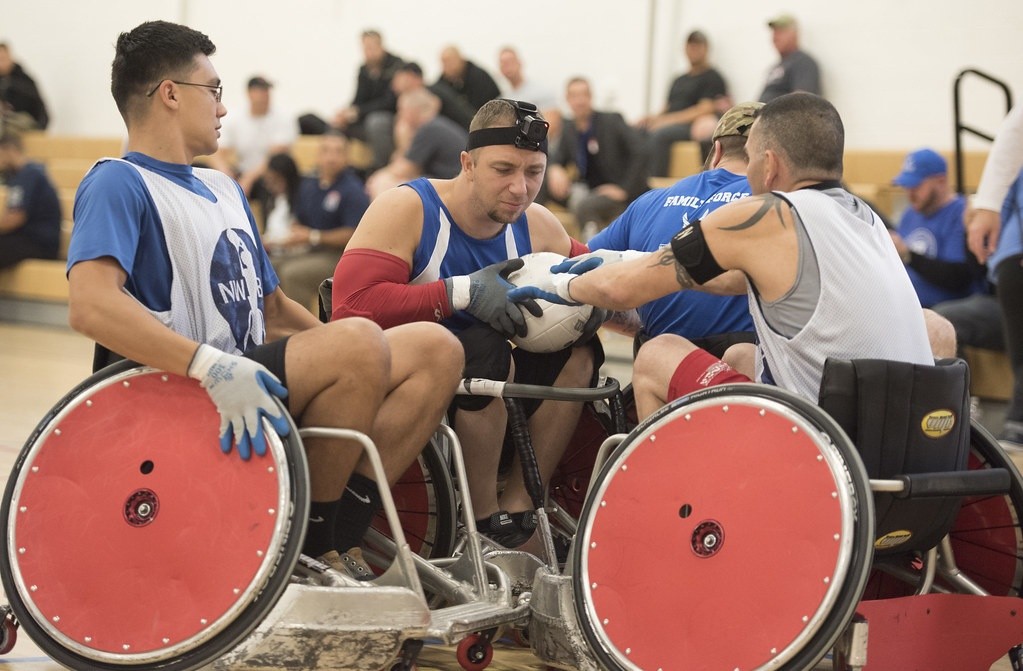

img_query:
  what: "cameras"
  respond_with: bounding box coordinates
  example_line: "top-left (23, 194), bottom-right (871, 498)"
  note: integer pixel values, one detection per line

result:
top-left (521, 115), bottom-right (549, 143)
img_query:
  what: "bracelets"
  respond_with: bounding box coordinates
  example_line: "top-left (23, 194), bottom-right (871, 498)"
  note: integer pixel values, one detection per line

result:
top-left (308, 229), bottom-right (321, 246)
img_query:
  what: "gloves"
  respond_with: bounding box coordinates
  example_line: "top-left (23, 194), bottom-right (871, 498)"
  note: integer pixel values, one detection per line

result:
top-left (186, 343), bottom-right (290, 459)
top-left (550, 248), bottom-right (653, 275)
top-left (442, 258), bottom-right (543, 339)
top-left (507, 272), bottom-right (585, 307)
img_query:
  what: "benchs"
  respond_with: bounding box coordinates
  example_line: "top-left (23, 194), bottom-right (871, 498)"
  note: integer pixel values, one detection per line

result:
top-left (0, 130), bottom-right (993, 331)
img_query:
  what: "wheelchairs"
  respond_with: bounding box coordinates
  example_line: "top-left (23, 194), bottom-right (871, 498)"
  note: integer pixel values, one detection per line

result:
top-left (0, 272), bottom-right (1023, 671)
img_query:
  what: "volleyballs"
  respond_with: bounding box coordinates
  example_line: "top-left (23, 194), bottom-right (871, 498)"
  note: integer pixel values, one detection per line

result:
top-left (507, 252), bottom-right (593, 353)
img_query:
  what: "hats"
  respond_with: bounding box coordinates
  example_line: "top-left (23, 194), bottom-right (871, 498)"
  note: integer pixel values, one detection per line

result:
top-left (893, 149), bottom-right (946, 188)
top-left (703, 101), bottom-right (766, 170)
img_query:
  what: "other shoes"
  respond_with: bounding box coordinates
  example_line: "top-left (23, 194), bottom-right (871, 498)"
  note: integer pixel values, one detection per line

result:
top-left (994, 421), bottom-right (1023, 453)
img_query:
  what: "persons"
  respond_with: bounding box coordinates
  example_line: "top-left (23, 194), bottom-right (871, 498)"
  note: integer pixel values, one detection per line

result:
top-left (190, 25), bottom-right (565, 314)
top-left (0, 129), bottom-right (61, 272)
top-left (553, 27), bottom-right (734, 241)
top-left (757, 14), bottom-right (822, 102)
top-left (327, 95), bottom-right (607, 565)
top-left (964, 103), bottom-right (1023, 262)
top-left (884, 145), bottom-right (1023, 445)
top-left (550, 88), bottom-right (938, 433)
top-left (0, 42), bottom-right (48, 143)
top-left (586, 101), bottom-right (958, 361)
top-left (66, 21), bottom-right (463, 578)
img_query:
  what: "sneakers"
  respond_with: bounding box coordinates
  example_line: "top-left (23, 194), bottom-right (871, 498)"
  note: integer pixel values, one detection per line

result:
top-left (457, 502), bottom-right (529, 548)
top-left (512, 510), bottom-right (570, 568)
top-left (343, 549), bottom-right (376, 581)
top-left (317, 550), bottom-right (353, 579)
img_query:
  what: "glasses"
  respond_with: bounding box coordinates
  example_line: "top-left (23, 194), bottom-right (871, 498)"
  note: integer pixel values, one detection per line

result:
top-left (147, 80), bottom-right (223, 103)
